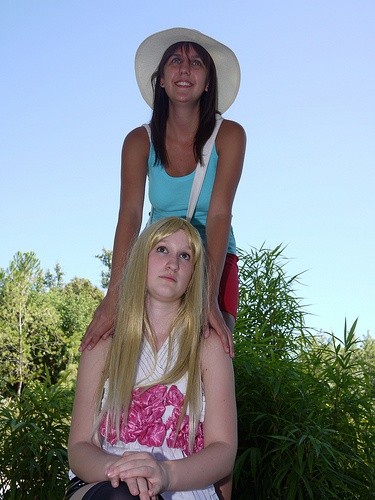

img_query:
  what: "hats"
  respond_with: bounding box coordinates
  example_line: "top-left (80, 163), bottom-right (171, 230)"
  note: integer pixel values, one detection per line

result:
top-left (135, 27), bottom-right (241, 116)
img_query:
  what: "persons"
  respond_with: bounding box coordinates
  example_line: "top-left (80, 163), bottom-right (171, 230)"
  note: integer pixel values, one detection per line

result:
top-left (76, 37), bottom-right (242, 500)
top-left (66, 216), bottom-right (239, 500)
